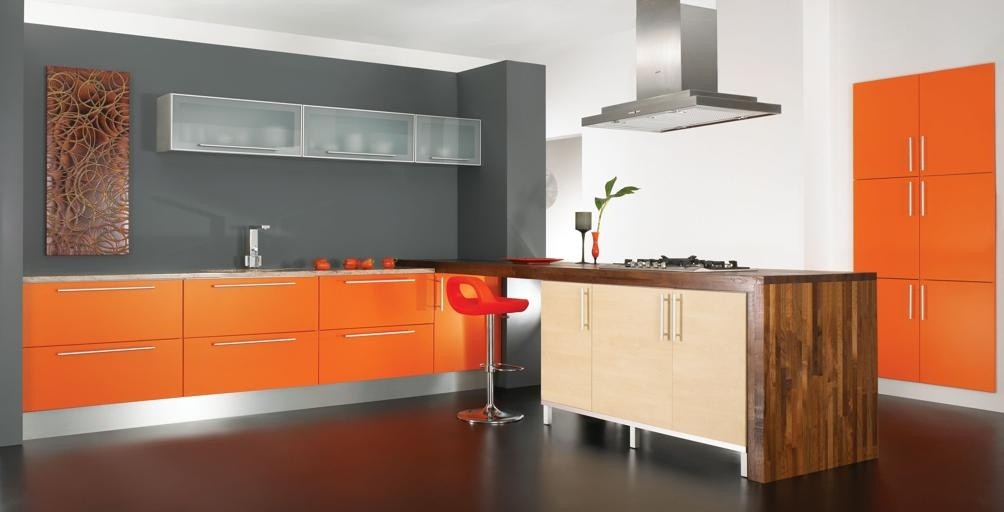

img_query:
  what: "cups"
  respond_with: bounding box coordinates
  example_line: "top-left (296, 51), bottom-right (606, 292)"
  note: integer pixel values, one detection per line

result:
top-left (382, 258), bottom-right (398, 269)
top-left (314, 258), bottom-right (375, 270)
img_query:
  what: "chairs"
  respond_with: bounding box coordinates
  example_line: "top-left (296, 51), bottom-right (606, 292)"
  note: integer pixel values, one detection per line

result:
top-left (445, 277), bottom-right (530, 427)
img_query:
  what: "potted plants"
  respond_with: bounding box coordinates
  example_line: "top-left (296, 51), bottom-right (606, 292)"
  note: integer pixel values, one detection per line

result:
top-left (590, 176), bottom-right (642, 264)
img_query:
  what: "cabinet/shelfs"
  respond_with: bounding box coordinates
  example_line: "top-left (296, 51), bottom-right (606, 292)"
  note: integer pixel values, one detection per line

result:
top-left (539, 280), bottom-right (592, 412)
top-left (852, 61), bottom-right (997, 396)
top-left (433, 272), bottom-right (503, 375)
top-left (183, 278), bottom-right (318, 397)
top-left (0, 279), bottom-right (182, 422)
top-left (590, 285), bottom-right (747, 449)
top-left (319, 274), bottom-right (433, 384)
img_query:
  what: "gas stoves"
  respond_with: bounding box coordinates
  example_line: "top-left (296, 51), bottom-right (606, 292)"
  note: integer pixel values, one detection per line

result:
top-left (619, 255), bottom-right (753, 274)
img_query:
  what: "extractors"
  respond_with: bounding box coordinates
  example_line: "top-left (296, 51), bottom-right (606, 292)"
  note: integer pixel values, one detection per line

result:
top-left (581, 0), bottom-right (782, 133)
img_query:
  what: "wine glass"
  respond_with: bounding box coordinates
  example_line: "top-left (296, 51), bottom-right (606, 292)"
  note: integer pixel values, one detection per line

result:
top-left (575, 211), bottom-right (592, 264)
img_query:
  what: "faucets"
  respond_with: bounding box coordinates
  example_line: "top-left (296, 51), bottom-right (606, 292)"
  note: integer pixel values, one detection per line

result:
top-left (242, 223), bottom-right (271, 269)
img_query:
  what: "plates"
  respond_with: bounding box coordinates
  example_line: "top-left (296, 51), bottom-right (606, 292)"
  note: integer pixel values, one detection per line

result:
top-left (495, 257), bottom-right (564, 265)
